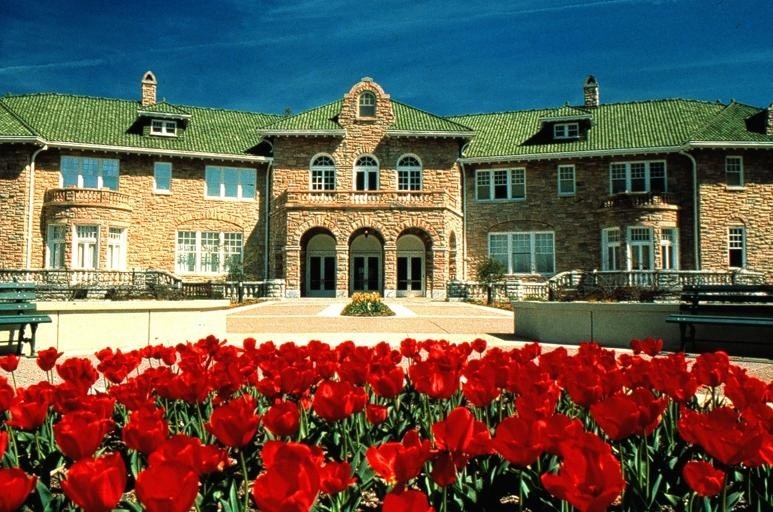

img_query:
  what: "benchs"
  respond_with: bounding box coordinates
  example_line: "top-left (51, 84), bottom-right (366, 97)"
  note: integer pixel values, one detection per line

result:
top-left (0, 283), bottom-right (51, 358)
top-left (665, 284), bottom-right (772, 355)
